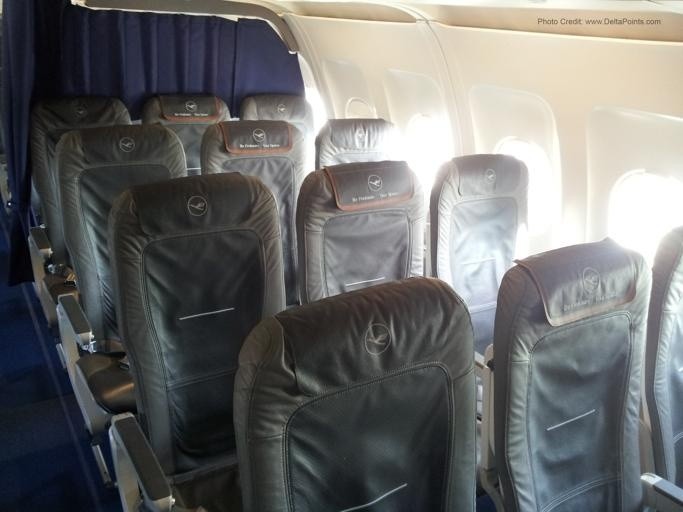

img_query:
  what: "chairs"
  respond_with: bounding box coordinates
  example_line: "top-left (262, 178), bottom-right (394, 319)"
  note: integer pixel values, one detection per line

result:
top-left (23, 87), bottom-right (683, 508)
